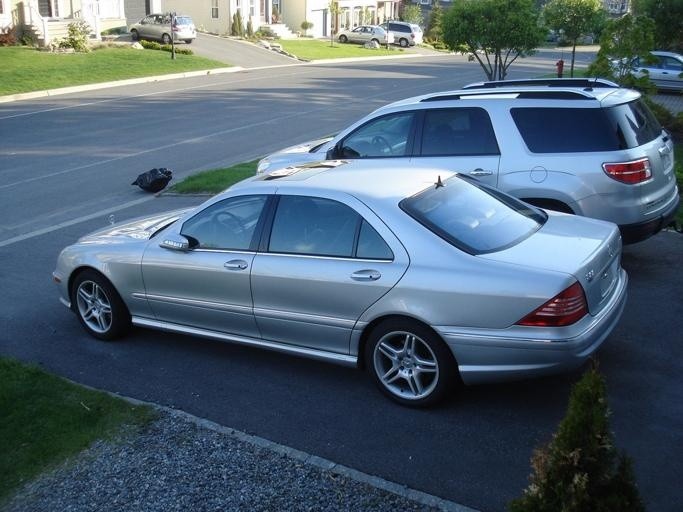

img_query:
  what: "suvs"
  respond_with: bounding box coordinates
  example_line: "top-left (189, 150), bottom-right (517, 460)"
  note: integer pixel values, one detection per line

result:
top-left (257, 78), bottom-right (679, 245)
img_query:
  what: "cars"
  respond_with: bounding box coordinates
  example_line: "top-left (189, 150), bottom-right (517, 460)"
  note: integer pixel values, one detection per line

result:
top-left (608, 51), bottom-right (683, 91)
top-left (379, 21), bottom-right (422, 48)
top-left (53, 161), bottom-right (630, 407)
top-left (337, 24), bottom-right (393, 45)
top-left (129, 12), bottom-right (197, 43)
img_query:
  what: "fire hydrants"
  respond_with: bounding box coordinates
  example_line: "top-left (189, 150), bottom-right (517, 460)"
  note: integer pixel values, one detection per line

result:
top-left (555, 60), bottom-right (564, 77)
